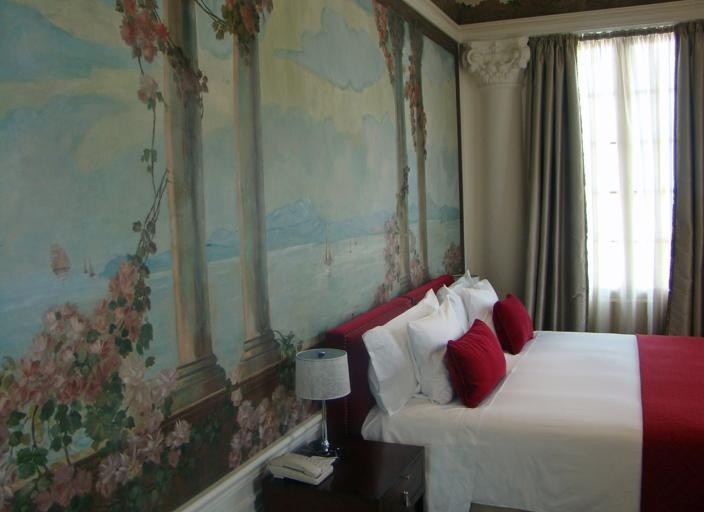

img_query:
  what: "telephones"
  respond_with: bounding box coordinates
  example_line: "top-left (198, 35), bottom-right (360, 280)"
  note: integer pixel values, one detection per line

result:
top-left (271, 453), bottom-right (334, 485)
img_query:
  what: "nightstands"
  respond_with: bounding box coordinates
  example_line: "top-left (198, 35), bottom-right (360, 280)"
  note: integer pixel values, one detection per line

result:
top-left (262, 438), bottom-right (427, 511)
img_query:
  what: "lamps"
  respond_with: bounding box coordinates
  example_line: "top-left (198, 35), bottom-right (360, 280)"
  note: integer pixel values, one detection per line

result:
top-left (294, 347), bottom-right (352, 456)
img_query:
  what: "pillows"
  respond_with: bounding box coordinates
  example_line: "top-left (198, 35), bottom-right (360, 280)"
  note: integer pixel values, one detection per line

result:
top-left (359, 268), bottom-right (535, 420)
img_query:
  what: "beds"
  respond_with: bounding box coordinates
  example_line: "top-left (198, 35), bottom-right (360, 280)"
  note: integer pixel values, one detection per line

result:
top-left (324, 274), bottom-right (704, 512)
